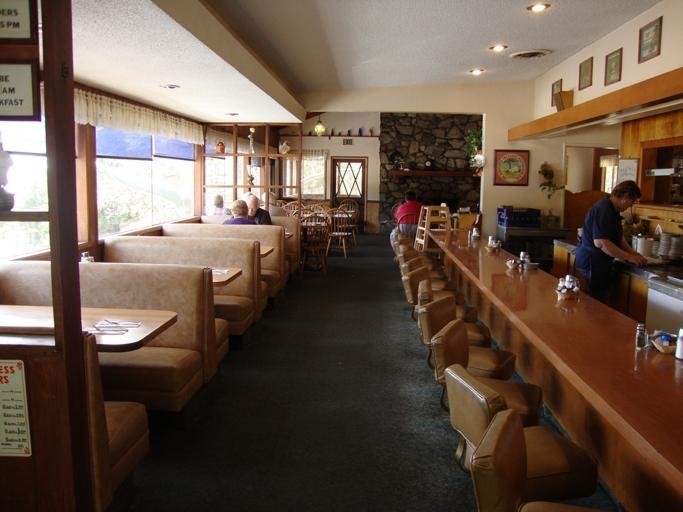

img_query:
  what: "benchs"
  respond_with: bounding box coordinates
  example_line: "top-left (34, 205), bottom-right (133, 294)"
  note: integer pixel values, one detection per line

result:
top-left (163, 222), bottom-right (291, 289)
top-left (162, 223), bottom-right (282, 297)
top-left (0, 326), bottom-right (150, 512)
top-left (7, 260), bottom-right (229, 384)
top-left (103, 235), bottom-right (255, 336)
top-left (118, 235), bottom-right (268, 322)
top-left (0, 260), bottom-right (206, 412)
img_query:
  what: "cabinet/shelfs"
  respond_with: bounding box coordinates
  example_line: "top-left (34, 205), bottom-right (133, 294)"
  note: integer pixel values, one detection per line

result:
top-left (551, 244), bottom-right (576, 280)
top-left (496, 225), bottom-right (570, 274)
top-left (618, 110), bottom-right (683, 240)
top-left (646, 288), bottom-right (683, 336)
top-left (608, 268), bottom-right (648, 325)
top-left (201, 123), bottom-right (301, 217)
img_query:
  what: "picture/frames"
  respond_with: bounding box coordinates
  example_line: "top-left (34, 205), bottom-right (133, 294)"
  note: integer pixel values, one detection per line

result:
top-left (579, 56), bottom-right (593, 91)
top-left (493, 148), bottom-right (530, 186)
top-left (603, 47), bottom-right (625, 87)
top-left (551, 79), bottom-right (562, 107)
top-left (636, 16), bottom-right (663, 64)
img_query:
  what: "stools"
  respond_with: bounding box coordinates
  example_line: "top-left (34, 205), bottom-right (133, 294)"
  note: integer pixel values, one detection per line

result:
top-left (414, 206), bottom-right (451, 252)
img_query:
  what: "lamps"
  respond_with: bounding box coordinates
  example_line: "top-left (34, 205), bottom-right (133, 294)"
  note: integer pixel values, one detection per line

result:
top-left (315, 113), bottom-right (326, 136)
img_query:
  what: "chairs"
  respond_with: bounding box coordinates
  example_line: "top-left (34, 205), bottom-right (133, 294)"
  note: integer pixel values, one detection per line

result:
top-left (444, 363), bottom-right (597, 500)
top-left (471, 409), bottom-right (605, 512)
top-left (304, 206), bottom-right (322, 213)
top-left (401, 266), bottom-right (479, 323)
top-left (301, 216), bottom-right (332, 274)
top-left (391, 201), bottom-right (406, 222)
top-left (396, 213), bottom-right (420, 246)
top-left (416, 278), bottom-right (490, 370)
top-left (397, 243), bottom-right (441, 271)
top-left (278, 200), bottom-right (288, 204)
top-left (338, 199), bottom-right (359, 242)
top-left (401, 257), bottom-right (465, 321)
top-left (399, 249), bottom-right (444, 280)
top-left (275, 201), bottom-right (282, 207)
top-left (318, 203), bottom-right (330, 211)
top-left (324, 209), bottom-right (353, 260)
top-left (334, 203), bottom-right (357, 245)
top-left (418, 294), bottom-right (515, 412)
top-left (284, 202), bottom-right (302, 211)
top-left (291, 201), bottom-right (305, 209)
top-left (431, 319), bottom-right (543, 426)
top-left (292, 209), bottom-right (316, 220)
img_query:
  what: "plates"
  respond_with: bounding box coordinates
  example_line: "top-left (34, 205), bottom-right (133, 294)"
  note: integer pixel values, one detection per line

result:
top-left (651, 241), bottom-right (660, 256)
top-left (632, 235), bottom-right (648, 252)
top-left (636, 238), bottom-right (654, 259)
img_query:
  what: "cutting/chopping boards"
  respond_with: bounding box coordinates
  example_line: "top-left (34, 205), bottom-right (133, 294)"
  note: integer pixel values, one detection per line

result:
top-left (624, 259), bottom-right (665, 267)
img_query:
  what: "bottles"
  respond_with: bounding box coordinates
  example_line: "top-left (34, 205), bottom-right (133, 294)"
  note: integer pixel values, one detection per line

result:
top-left (520, 252), bottom-right (528, 260)
top-left (473, 228), bottom-right (479, 237)
top-left (81, 252), bottom-right (94, 262)
top-left (636, 324), bottom-right (645, 351)
top-left (488, 236), bottom-right (496, 247)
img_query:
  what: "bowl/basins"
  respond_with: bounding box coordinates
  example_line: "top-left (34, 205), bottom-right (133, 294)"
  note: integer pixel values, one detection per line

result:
top-left (667, 237), bottom-right (683, 260)
top-left (472, 235), bottom-right (480, 239)
top-left (656, 232), bottom-right (674, 259)
top-left (484, 246), bottom-right (498, 252)
top-left (385, 164), bottom-right (395, 170)
top-left (523, 263), bottom-right (540, 271)
top-left (555, 290), bottom-right (579, 300)
top-left (506, 263), bottom-right (520, 270)
top-left (650, 335), bottom-right (677, 353)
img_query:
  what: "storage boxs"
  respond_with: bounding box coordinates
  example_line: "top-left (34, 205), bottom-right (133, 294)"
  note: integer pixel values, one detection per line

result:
top-left (497, 205), bottom-right (541, 228)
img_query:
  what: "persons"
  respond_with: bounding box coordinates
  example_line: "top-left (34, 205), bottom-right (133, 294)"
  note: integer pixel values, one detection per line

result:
top-left (245, 195), bottom-right (272, 226)
top-left (208, 195), bottom-right (230, 217)
top-left (390, 190), bottom-right (425, 255)
top-left (573, 180), bottom-right (647, 299)
top-left (262, 192), bottom-right (286, 216)
top-left (223, 200), bottom-right (257, 225)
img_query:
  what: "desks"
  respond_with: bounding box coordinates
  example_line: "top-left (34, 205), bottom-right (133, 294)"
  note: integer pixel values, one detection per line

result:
top-left (1, 305), bottom-right (180, 353)
